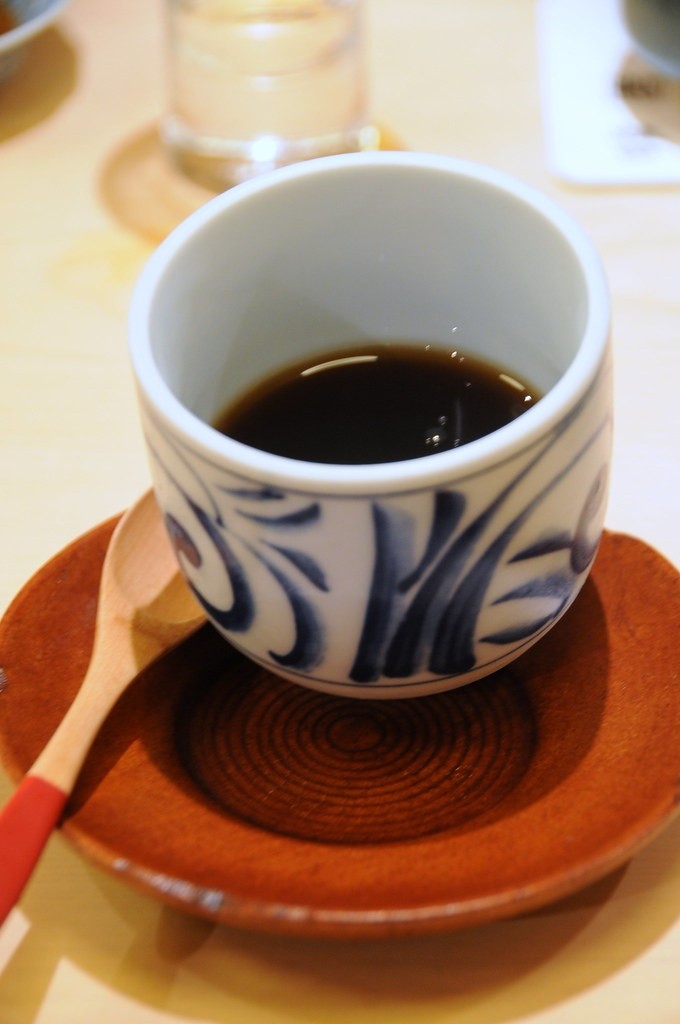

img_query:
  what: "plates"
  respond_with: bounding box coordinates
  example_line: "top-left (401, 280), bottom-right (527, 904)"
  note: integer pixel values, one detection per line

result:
top-left (0, 509), bottom-right (678, 944)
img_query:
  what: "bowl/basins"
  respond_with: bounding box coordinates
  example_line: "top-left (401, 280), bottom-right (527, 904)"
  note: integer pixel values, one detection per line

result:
top-left (0, 0), bottom-right (68, 60)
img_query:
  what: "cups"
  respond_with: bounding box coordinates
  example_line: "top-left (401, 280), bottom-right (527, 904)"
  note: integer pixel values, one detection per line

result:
top-left (163, 0), bottom-right (368, 197)
top-left (130, 150), bottom-right (615, 696)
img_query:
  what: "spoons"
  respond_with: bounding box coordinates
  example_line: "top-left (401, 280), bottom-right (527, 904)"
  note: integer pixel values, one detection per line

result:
top-left (0, 487), bottom-right (210, 923)
